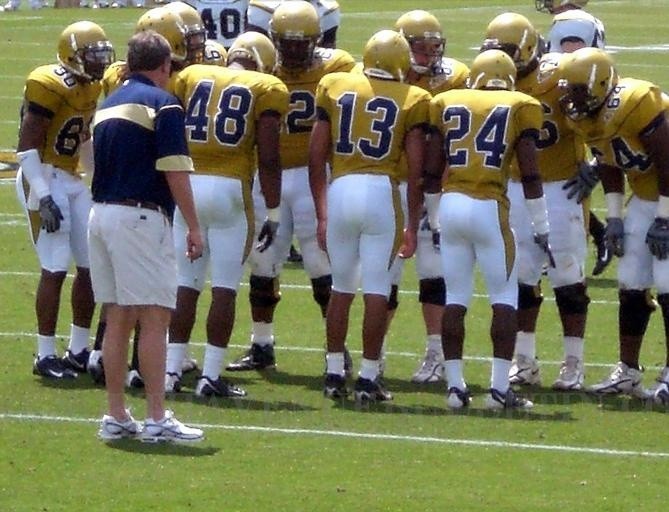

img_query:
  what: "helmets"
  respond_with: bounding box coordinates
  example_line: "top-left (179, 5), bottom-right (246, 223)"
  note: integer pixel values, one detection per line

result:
top-left (559, 49), bottom-right (618, 122)
top-left (225, 31), bottom-right (278, 74)
top-left (397, 9), bottom-right (444, 79)
top-left (136, 2), bottom-right (207, 72)
top-left (363, 29), bottom-right (412, 82)
top-left (56, 21), bottom-right (114, 84)
top-left (535, 0), bottom-right (587, 15)
top-left (468, 50), bottom-right (516, 91)
top-left (483, 12), bottom-right (539, 67)
top-left (270, 1), bottom-right (322, 40)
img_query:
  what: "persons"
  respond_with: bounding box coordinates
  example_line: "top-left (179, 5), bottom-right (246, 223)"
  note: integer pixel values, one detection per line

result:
top-left (11, 4), bottom-right (666, 415)
top-left (86, 29), bottom-right (206, 448)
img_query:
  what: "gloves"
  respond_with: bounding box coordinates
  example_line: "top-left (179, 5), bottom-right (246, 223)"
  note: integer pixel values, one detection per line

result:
top-left (257, 219), bottom-right (279, 252)
top-left (432, 228), bottom-right (441, 253)
top-left (535, 232), bottom-right (550, 253)
top-left (563, 164), bottom-right (598, 205)
top-left (603, 218), bottom-right (624, 257)
top-left (644, 219), bottom-right (668, 260)
top-left (38, 195), bottom-right (64, 234)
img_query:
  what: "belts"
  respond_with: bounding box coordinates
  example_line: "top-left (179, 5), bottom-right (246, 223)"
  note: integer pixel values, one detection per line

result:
top-left (95, 198), bottom-right (159, 213)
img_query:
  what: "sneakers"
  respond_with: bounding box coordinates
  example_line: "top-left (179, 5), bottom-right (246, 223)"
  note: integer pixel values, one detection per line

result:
top-left (31, 349), bottom-right (144, 390)
top-left (487, 388), bottom-right (533, 411)
top-left (4, 3), bottom-right (43, 12)
top-left (590, 360), bottom-right (643, 393)
top-left (197, 376), bottom-right (246, 398)
top-left (288, 246), bottom-right (302, 262)
top-left (79, 1), bottom-right (145, 8)
top-left (592, 231), bottom-right (614, 275)
top-left (509, 357), bottom-right (541, 385)
top-left (99, 410), bottom-right (143, 439)
top-left (638, 368), bottom-right (668, 398)
top-left (411, 358), bottom-right (445, 382)
top-left (225, 341), bottom-right (276, 371)
top-left (144, 412), bottom-right (205, 444)
top-left (447, 384), bottom-right (472, 407)
top-left (552, 358), bottom-right (586, 390)
top-left (165, 356), bottom-right (195, 392)
top-left (322, 352), bottom-right (394, 403)
